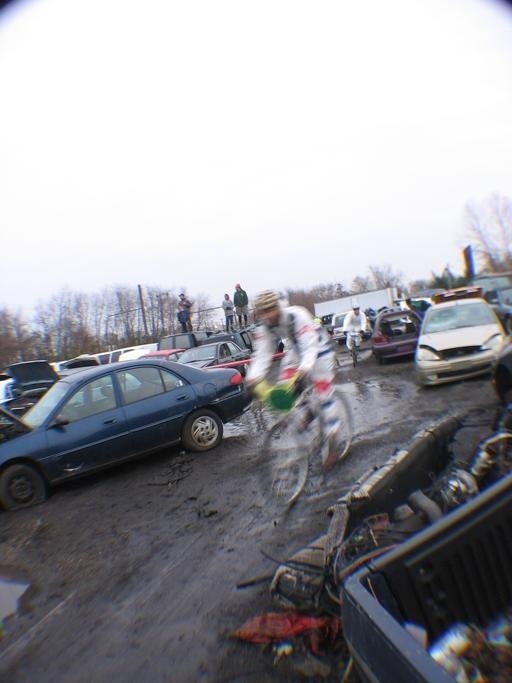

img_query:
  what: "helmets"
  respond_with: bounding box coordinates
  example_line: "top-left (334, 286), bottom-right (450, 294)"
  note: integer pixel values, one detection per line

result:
top-left (255, 291), bottom-right (278, 311)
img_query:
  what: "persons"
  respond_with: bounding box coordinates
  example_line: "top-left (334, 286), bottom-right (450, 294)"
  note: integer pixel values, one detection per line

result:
top-left (177, 292), bottom-right (193, 332)
top-left (234, 283), bottom-right (250, 328)
top-left (221, 293), bottom-right (236, 333)
top-left (343, 303), bottom-right (367, 356)
top-left (245, 288), bottom-right (340, 469)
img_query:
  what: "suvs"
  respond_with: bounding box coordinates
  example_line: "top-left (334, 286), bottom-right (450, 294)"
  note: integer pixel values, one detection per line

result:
top-left (321, 312), bottom-right (336, 331)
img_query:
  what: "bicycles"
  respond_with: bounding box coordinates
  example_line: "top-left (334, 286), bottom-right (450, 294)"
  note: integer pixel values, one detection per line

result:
top-left (252, 378), bottom-right (356, 509)
top-left (342, 330), bottom-right (366, 367)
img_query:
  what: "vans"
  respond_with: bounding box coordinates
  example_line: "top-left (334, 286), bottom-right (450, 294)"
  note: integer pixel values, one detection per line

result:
top-left (331, 310), bottom-right (372, 342)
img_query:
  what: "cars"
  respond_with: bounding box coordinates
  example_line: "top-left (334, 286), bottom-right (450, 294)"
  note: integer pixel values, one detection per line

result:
top-left (371, 286), bottom-right (512, 333)
top-left (413, 295), bottom-right (507, 385)
top-left (0, 334), bottom-right (255, 408)
top-left (373, 309), bottom-right (423, 360)
top-left (0, 363), bottom-right (247, 510)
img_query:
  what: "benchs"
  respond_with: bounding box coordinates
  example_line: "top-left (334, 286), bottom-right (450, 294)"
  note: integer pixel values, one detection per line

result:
top-left (267, 410), bottom-right (467, 617)
